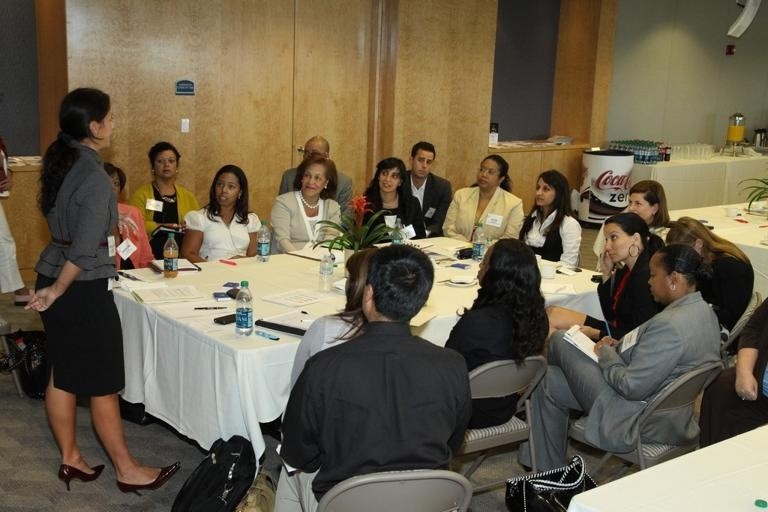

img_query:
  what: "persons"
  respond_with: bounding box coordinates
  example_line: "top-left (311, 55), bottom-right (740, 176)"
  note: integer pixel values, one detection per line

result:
top-left (23, 88), bottom-right (181, 497)
top-left (0, 136), bottom-right (36, 306)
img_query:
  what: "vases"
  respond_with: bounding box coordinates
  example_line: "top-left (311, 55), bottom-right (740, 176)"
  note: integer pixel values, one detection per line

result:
top-left (344, 250), bottom-right (354, 276)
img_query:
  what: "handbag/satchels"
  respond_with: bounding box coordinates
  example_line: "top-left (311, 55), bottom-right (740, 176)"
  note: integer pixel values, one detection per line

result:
top-left (170, 435), bottom-right (276, 512)
top-left (503, 455), bottom-right (597, 511)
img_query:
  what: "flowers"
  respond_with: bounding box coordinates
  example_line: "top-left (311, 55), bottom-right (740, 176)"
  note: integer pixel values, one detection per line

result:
top-left (310, 192), bottom-right (408, 251)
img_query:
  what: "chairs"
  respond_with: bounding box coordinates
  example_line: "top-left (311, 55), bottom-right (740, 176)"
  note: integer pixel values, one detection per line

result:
top-left (454, 355), bottom-right (550, 495)
top-left (315, 468), bottom-right (474, 511)
top-left (566, 361), bottom-right (724, 471)
top-left (720, 289), bottom-right (763, 369)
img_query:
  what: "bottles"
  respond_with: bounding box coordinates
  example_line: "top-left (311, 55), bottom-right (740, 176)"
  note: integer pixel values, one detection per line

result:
top-left (753, 129), bottom-right (766, 151)
top-left (318, 253), bottom-right (333, 293)
top-left (254, 220), bottom-right (271, 263)
top-left (391, 225), bottom-right (401, 245)
top-left (608, 136), bottom-right (671, 165)
top-left (235, 280), bottom-right (254, 335)
top-left (163, 233), bottom-right (179, 279)
top-left (472, 223), bottom-right (488, 262)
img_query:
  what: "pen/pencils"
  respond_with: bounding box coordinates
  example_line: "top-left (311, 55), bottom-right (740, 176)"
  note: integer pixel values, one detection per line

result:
top-left (194, 307), bottom-right (226, 310)
top-left (219, 260), bottom-right (236, 266)
top-left (117, 271), bottom-right (141, 281)
top-left (255, 330), bottom-right (280, 340)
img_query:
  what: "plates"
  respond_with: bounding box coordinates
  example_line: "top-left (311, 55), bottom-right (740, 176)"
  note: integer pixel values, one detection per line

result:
top-left (445, 280), bottom-right (478, 287)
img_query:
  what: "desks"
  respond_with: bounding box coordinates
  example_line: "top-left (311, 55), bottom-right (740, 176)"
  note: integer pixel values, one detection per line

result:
top-left (659, 199), bottom-right (768, 299)
top-left (624, 145), bottom-right (768, 215)
top-left (561, 422), bottom-right (768, 512)
top-left (105, 234), bottom-right (606, 479)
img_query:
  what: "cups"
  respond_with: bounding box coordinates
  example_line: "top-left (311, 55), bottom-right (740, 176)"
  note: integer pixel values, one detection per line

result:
top-left (670, 143), bottom-right (715, 162)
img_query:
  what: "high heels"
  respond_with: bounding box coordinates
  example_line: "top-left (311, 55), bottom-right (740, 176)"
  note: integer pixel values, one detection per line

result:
top-left (58, 463), bottom-right (103, 491)
top-left (116, 462), bottom-right (180, 497)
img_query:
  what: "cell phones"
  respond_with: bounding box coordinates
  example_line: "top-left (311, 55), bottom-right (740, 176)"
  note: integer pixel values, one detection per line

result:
top-left (214, 314), bottom-right (236, 325)
